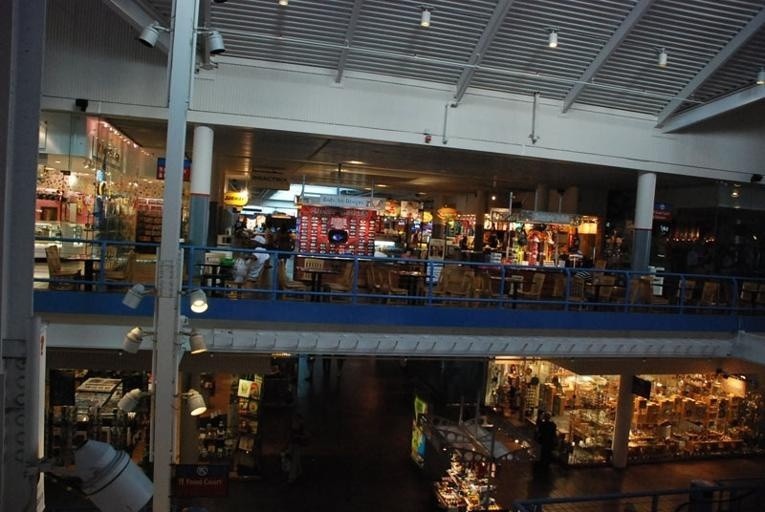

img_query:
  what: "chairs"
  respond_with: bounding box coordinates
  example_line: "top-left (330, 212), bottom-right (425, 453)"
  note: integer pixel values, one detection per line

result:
top-left (47, 245), bottom-right (764, 315)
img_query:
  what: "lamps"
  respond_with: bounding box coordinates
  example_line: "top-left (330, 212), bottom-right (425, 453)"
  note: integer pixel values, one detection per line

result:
top-left (546, 26), bottom-right (561, 48)
top-left (757, 68), bottom-right (763, 84)
top-left (138, 22), bottom-right (170, 49)
top-left (419, 2), bottom-right (436, 28)
top-left (180, 328), bottom-right (207, 355)
top-left (197, 29), bottom-right (226, 55)
top-left (117, 388), bottom-right (154, 414)
top-left (655, 46), bottom-right (668, 66)
top-left (22, 438), bottom-right (154, 512)
top-left (121, 328), bottom-right (154, 355)
top-left (175, 388), bottom-right (207, 416)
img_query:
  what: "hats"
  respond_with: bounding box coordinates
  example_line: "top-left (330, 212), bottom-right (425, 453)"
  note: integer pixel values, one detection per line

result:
top-left (250, 235), bottom-right (266, 245)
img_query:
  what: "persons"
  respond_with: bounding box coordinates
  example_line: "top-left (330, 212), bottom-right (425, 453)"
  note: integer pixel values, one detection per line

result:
top-left (538, 411), bottom-right (557, 458)
top-left (535, 408), bottom-right (545, 460)
top-left (209, 200), bottom-right (296, 291)
